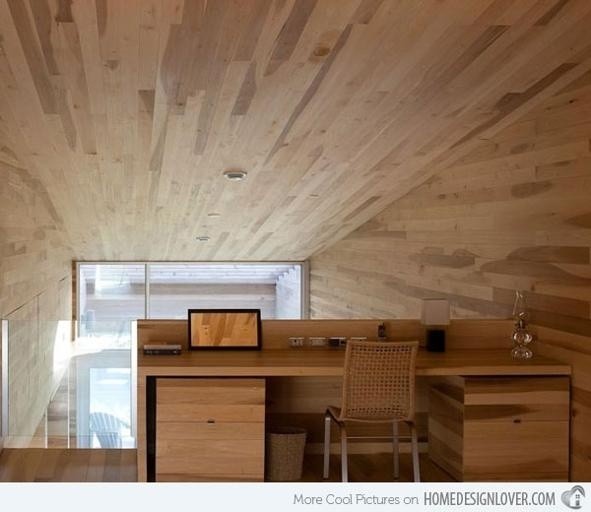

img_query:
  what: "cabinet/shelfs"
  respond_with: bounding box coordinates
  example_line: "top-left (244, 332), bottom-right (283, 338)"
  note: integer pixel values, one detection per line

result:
top-left (155, 377), bottom-right (265, 486)
top-left (429, 375), bottom-right (571, 483)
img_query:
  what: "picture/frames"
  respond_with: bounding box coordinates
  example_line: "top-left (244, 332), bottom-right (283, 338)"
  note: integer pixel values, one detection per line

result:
top-left (186, 308), bottom-right (263, 350)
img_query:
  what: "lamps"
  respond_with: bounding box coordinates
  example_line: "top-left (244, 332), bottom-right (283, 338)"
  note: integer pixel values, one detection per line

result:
top-left (420, 298), bottom-right (451, 353)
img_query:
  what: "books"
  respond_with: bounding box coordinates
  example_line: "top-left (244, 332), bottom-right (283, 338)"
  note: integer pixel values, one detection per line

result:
top-left (144, 349), bottom-right (181, 355)
top-left (144, 345), bottom-right (182, 350)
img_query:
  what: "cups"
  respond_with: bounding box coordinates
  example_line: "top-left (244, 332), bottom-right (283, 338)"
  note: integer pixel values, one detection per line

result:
top-left (377, 320), bottom-right (386, 339)
top-left (288, 337), bottom-right (305, 347)
top-left (329, 337), bottom-right (347, 347)
top-left (350, 336), bottom-right (367, 342)
top-left (309, 336), bottom-right (327, 346)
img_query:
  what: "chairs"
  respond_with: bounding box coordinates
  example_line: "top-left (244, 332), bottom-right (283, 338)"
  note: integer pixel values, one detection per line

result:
top-left (323, 341), bottom-right (421, 483)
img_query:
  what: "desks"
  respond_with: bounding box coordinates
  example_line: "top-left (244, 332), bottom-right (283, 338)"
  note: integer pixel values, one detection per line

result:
top-left (137, 344), bottom-right (572, 483)
